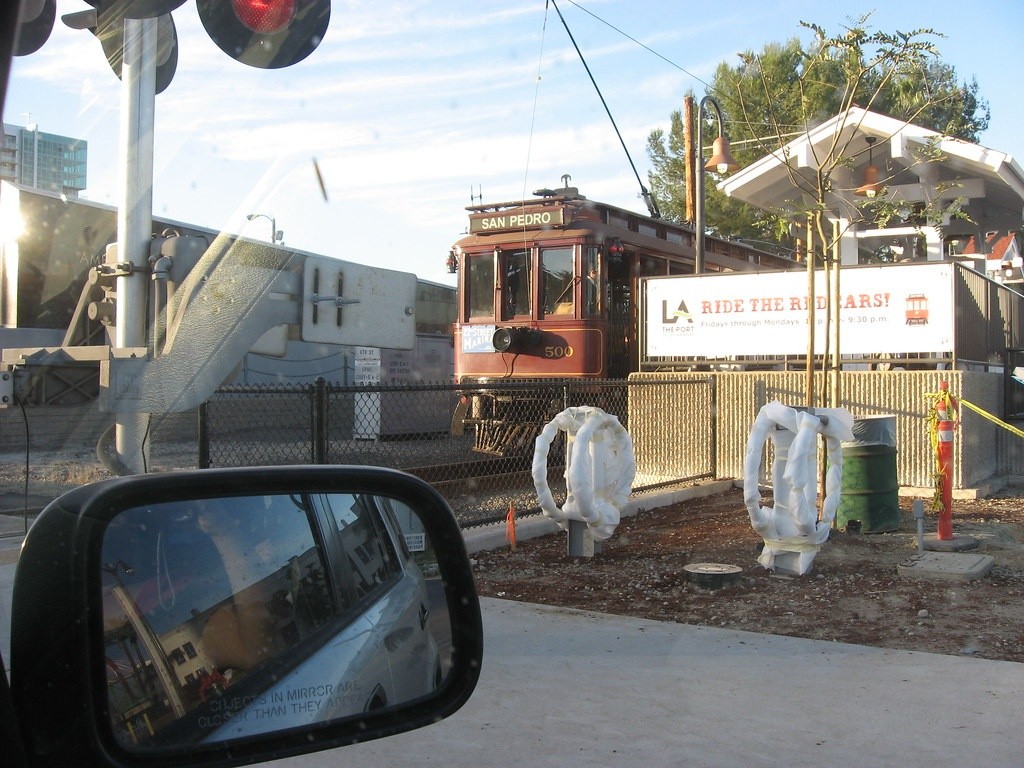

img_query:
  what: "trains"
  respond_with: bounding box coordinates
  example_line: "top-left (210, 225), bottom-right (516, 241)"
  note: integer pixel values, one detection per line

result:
top-left (445, 174), bottom-right (815, 464)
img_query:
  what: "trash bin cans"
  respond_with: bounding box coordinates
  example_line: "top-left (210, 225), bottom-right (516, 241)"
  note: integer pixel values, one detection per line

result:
top-left (825, 414), bottom-right (900, 534)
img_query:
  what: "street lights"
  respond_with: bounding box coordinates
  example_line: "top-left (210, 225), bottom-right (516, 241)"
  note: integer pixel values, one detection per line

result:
top-left (694, 95), bottom-right (741, 277)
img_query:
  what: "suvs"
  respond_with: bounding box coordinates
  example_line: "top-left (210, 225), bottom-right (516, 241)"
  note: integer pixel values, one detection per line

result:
top-left (93, 488), bottom-right (444, 748)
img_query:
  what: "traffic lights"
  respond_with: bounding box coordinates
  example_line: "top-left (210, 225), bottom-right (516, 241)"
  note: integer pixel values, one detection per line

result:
top-left (195, 0), bottom-right (333, 69)
